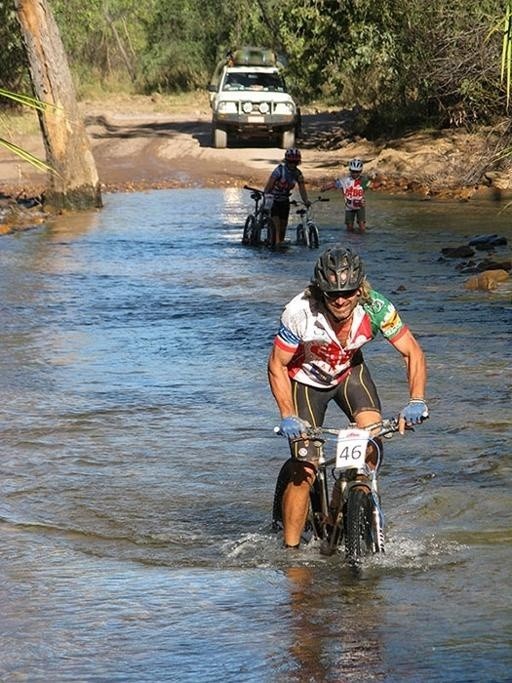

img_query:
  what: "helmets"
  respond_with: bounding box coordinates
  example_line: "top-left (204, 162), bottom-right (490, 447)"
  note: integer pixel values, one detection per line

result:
top-left (284, 149), bottom-right (301, 162)
top-left (349, 157), bottom-right (363, 171)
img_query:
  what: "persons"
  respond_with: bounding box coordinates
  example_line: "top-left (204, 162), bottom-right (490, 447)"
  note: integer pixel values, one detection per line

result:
top-left (264, 149), bottom-right (312, 244)
top-left (268, 245), bottom-right (428, 551)
top-left (321, 159), bottom-right (382, 232)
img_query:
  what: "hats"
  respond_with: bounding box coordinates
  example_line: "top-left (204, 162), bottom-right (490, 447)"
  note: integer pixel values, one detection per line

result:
top-left (312, 244), bottom-right (363, 292)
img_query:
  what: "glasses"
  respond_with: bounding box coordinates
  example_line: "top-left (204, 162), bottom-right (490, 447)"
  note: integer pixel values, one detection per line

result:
top-left (322, 290), bottom-right (358, 299)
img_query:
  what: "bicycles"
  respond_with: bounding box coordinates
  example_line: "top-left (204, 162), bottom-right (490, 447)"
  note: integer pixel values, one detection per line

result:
top-left (268, 412), bottom-right (430, 581)
top-left (284, 195), bottom-right (331, 249)
top-left (240, 183), bottom-right (290, 249)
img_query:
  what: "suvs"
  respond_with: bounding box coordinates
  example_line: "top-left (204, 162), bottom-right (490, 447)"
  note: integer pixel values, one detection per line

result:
top-left (206, 48), bottom-right (302, 153)
